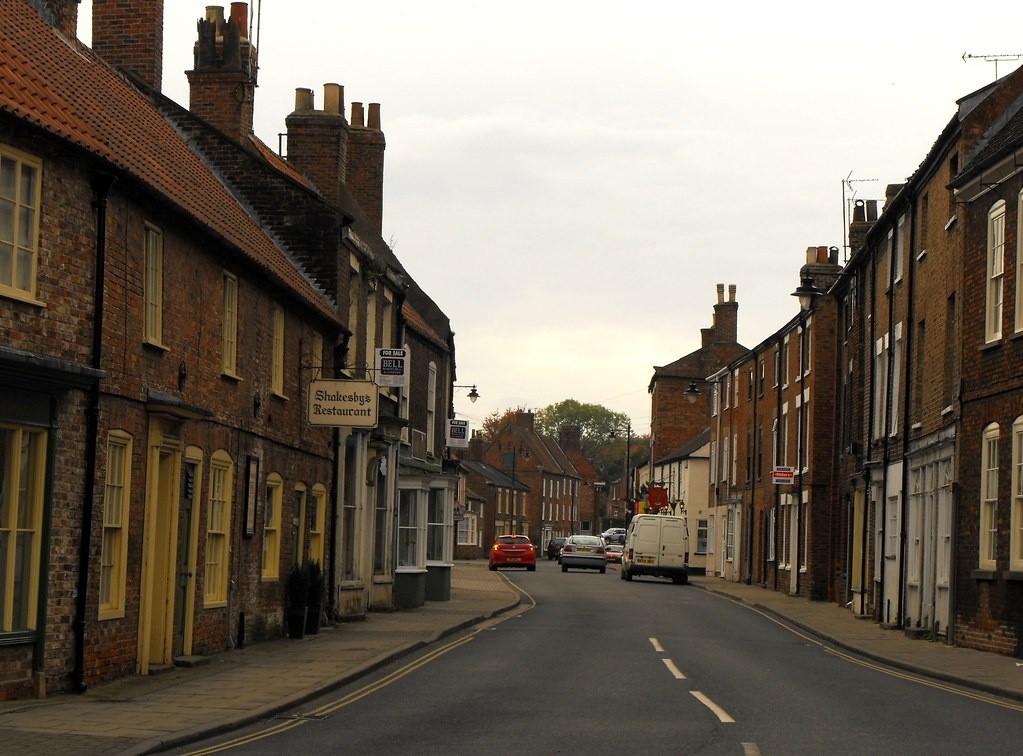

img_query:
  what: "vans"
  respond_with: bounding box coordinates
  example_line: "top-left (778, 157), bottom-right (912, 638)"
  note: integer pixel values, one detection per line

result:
top-left (621, 513), bottom-right (689, 584)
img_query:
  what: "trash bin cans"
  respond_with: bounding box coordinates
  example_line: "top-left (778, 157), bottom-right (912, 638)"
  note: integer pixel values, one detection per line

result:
top-left (395, 569), bottom-right (428, 607)
top-left (425, 564), bottom-right (453, 600)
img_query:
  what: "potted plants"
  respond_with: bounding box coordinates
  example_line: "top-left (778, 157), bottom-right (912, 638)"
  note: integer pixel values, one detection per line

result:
top-left (286, 558), bottom-right (325, 640)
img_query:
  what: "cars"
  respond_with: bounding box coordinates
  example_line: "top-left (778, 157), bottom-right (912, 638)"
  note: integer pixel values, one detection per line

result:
top-left (488, 534), bottom-right (536, 572)
top-left (562, 534), bottom-right (607, 574)
top-left (547, 527), bottom-right (629, 563)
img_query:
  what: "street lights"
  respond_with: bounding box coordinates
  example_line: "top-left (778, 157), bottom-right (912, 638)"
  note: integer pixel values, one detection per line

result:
top-left (509, 445), bottom-right (531, 538)
top-left (570, 474), bottom-right (591, 535)
top-left (608, 423), bottom-right (631, 527)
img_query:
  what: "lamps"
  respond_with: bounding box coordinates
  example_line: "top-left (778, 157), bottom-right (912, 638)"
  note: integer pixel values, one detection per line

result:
top-left (450, 384), bottom-right (480, 403)
top-left (791, 269), bottom-right (859, 312)
top-left (679, 500), bottom-right (686, 515)
top-left (682, 378), bottom-right (723, 404)
